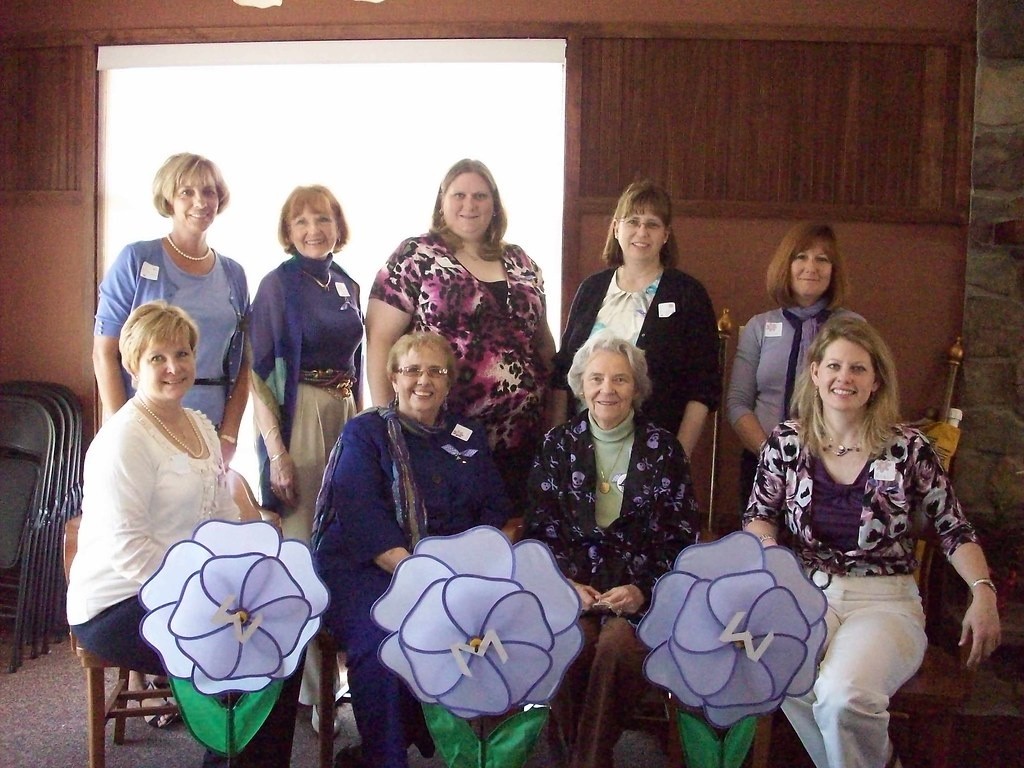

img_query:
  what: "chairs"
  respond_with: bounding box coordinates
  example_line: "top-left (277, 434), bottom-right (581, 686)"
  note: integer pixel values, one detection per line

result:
top-left (62, 502), bottom-right (227, 768)
top-left (890, 523), bottom-right (1018, 768)
top-left (1, 377), bottom-right (82, 672)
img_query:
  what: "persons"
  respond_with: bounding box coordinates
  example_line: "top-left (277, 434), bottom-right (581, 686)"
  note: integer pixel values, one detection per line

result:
top-left (523, 328), bottom-right (701, 768)
top-left (365, 158), bottom-right (556, 517)
top-left (743, 317), bottom-right (1001, 768)
top-left (549, 181), bottom-right (724, 462)
top-left (92, 153), bottom-right (250, 468)
top-left (247, 183), bottom-right (365, 546)
top-left (66, 298), bottom-right (308, 768)
top-left (311, 331), bottom-right (510, 768)
top-left (727, 221), bottom-right (867, 512)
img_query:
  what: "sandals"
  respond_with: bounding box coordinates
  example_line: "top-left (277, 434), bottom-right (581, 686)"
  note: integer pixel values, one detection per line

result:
top-left (140, 682), bottom-right (179, 728)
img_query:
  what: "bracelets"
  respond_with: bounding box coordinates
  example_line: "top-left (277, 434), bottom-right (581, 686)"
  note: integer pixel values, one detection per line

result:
top-left (220, 435), bottom-right (236, 445)
top-left (271, 451), bottom-right (286, 462)
top-left (971, 578), bottom-right (997, 594)
top-left (760, 534), bottom-right (775, 542)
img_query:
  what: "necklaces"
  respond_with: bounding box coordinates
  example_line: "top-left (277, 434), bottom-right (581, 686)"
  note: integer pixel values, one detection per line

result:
top-left (135, 393), bottom-right (204, 458)
top-left (304, 270), bottom-right (331, 287)
top-left (829, 437), bottom-right (859, 456)
top-left (595, 438), bottom-right (624, 494)
top-left (167, 233), bottom-right (210, 260)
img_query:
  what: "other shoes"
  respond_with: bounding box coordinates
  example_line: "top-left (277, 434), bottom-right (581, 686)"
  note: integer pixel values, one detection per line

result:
top-left (312, 704), bottom-right (339, 739)
top-left (204, 749), bottom-right (219, 768)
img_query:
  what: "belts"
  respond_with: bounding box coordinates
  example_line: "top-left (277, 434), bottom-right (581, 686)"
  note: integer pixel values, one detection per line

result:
top-left (194, 378), bottom-right (228, 385)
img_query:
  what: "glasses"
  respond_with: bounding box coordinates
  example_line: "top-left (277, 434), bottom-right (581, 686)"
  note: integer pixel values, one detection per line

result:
top-left (623, 219), bottom-right (666, 232)
top-left (393, 366), bottom-right (453, 378)
